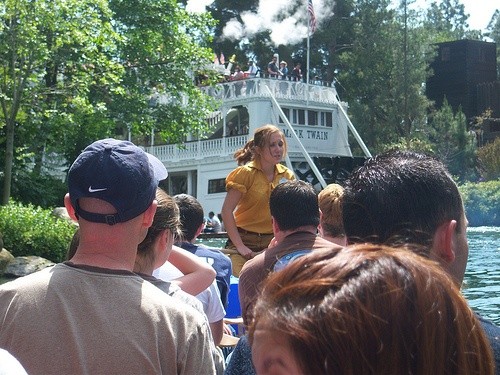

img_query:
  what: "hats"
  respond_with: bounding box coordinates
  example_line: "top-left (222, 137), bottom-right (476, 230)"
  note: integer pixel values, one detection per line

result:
top-left (67, 138), bottom-right (168, 225)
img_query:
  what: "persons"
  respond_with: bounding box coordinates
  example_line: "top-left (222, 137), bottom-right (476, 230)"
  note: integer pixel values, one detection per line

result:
top-left (0, 138), bottom-right (218, 375)
top-left (131, 186), bottom-right (226, 375)
top-left (200, 146), bottom-right (500, 375)
top-left (195, 56), bottom-right (302, 82)
top-left (170, 193), bottom-right (235, 359)
top-left (69, 227), bottom-right (217, 297)
top-left (221, 124), bottom-right (297, 280)
top-left (152, 259), bottom-right (226, 348)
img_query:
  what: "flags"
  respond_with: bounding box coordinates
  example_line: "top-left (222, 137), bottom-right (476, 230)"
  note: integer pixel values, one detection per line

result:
top-left (307, 0), bottom-right (316, 32)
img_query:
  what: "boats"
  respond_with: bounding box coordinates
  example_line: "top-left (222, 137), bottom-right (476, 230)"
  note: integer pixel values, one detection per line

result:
top-left (104, 74), bottom-right (374, 239)
